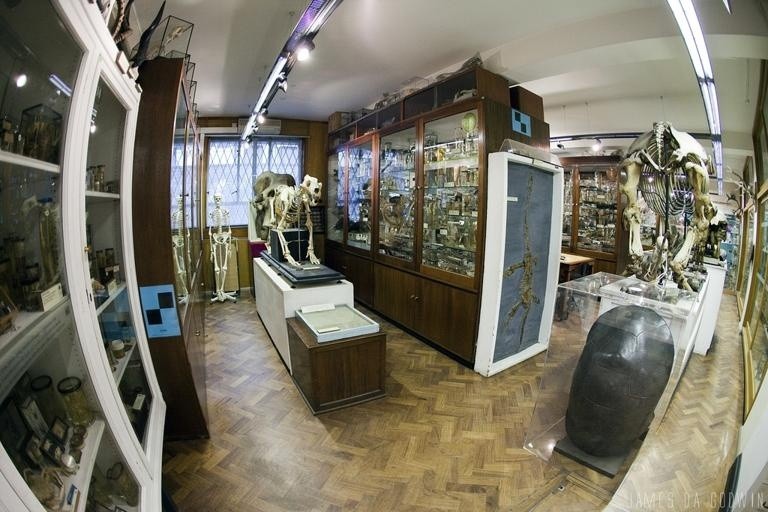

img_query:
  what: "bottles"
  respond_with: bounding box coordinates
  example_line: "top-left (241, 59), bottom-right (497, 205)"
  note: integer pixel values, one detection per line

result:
top-left (56, 375), bottom-right (96, 428)
top-left (95, 249), bottom-right (105, 268)
top-left (105, 247), bottom-right (115, 267)
top-left (107, 461), bottom-right (139, 508)
top-left (85, 164), bottom-right (106, 193)
top-left (0, 235), bottom-right (40, 306)
top-left (111, 339), bottom-right (125, 359)
top-left (30, 375), bottom-right (58, 425)
